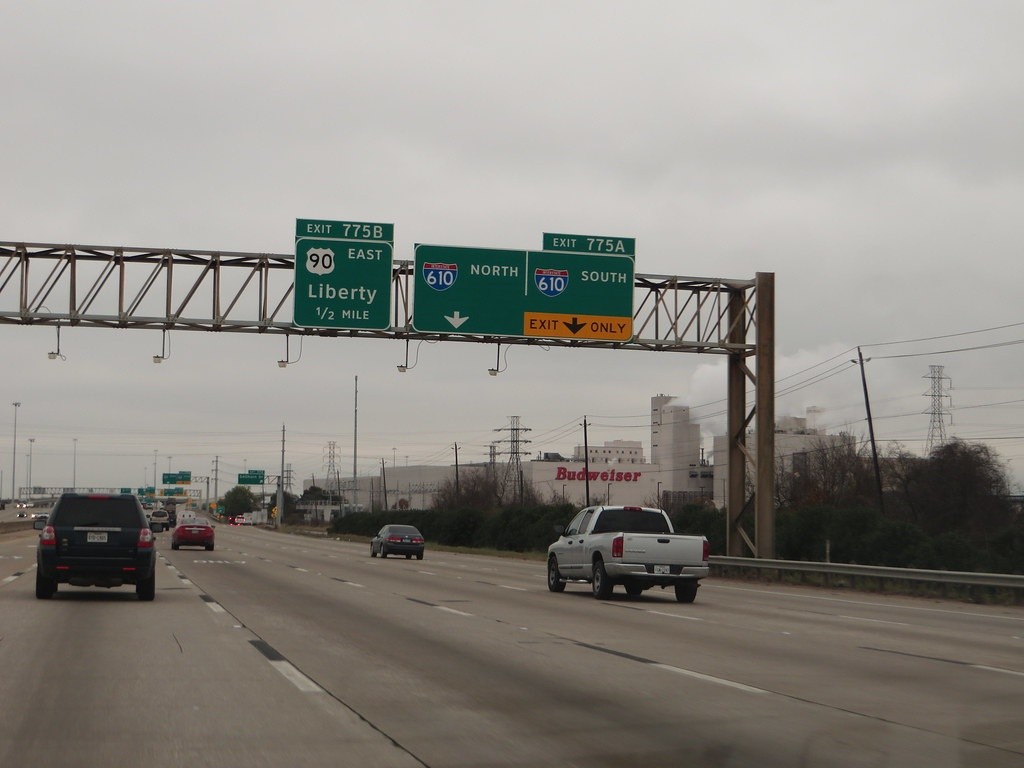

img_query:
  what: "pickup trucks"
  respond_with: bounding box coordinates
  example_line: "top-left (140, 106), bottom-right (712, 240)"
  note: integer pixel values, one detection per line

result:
top-left (548, 504), bottom-right (710, 605)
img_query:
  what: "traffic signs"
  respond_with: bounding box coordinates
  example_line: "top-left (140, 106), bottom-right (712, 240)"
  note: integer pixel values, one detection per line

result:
top-left (412, 232), bottom-right (635, 343)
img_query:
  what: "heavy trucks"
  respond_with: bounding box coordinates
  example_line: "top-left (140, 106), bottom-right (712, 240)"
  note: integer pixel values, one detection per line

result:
top-left (165, 501), bottom-right (176, 512)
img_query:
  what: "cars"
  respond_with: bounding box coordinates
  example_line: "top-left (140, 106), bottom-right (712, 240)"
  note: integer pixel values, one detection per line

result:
top-left (17, 502), bottom-right (34, 509)
top-left (371, 525), bottom-right (424, 560)
top-left (171, 518), bottom-right (215, 551)
top-left (18, 511), bottom-right (27, 517)
top-left (32, 512), bottom-right (49, 518)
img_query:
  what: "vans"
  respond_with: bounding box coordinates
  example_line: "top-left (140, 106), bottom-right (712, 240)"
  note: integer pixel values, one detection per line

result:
top-left (177, 511), bottom-right (195, 526)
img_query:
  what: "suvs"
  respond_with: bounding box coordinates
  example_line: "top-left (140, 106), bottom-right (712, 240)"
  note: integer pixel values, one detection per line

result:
top-left (145, 503), bottom-right (153, 509)
top-left (229, 515), bottom-right (246, 525)
top-left (147, 510), bottom-right (170, 531)
top-left (34, 494), bottom-right (163, 599)
top-left (169, 514), bottom-right (176, 526)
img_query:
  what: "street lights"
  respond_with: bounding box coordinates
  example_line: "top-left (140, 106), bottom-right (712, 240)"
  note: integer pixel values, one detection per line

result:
top-left (73, 437), bottom-right (78, 485)
top-left (12, 402), bottom-right (20, 499)
top-left (29, 438), bottom-right (35, 502)
